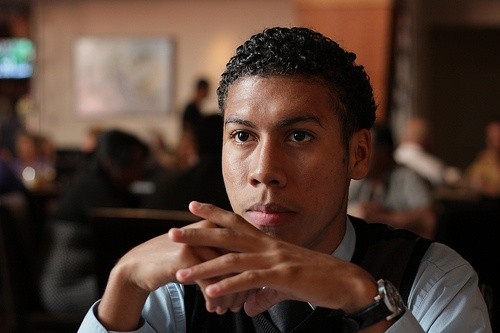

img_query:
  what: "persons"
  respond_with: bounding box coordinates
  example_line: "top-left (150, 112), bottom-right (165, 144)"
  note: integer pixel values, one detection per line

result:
top-left (349, 125), bottom-right (436, 238)
top-left (468, 119), bottom-right (500, 196)
top-left (77, 27), bottom-right (493, 333)
top-left (0, 79), bottom-right (224, 316)
top-left (393, 119), bottom-right (460, 197)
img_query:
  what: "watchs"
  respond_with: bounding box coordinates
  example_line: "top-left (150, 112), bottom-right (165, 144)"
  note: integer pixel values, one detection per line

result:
top-left (342, 278), bottom-right (405, 333)
